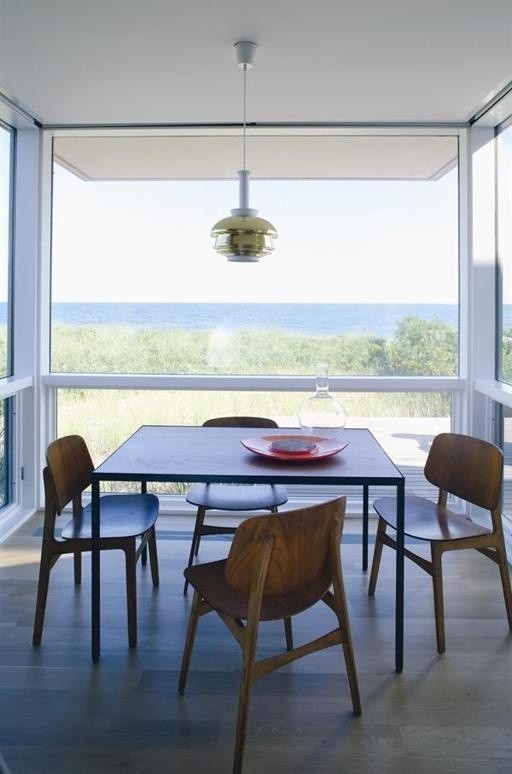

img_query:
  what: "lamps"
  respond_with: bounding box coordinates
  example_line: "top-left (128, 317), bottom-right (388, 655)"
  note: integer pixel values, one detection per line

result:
top-left (209, 40), bottom-right (277, 265)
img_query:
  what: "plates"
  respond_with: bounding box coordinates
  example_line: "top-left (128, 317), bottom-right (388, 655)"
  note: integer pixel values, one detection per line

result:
top-left (239, 434), bottom-right (348, 462)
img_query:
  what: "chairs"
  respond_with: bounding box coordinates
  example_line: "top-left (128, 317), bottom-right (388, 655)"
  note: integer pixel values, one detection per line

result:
top-left (183, 417), bottom-right (282, 592)
top-left (33, 436), bottom-right (160, 647)
top-left (368, 433), bottom-right (512, 655)
top-left (178, 497), bottom-right (361, 772)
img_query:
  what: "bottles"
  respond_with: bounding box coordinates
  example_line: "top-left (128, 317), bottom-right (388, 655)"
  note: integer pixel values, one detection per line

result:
top-left (298, 361), bottom-right (346, 437)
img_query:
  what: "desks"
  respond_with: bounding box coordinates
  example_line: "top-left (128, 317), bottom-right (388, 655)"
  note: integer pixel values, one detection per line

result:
top-left (91, 425), bottom-right (405, 673)
